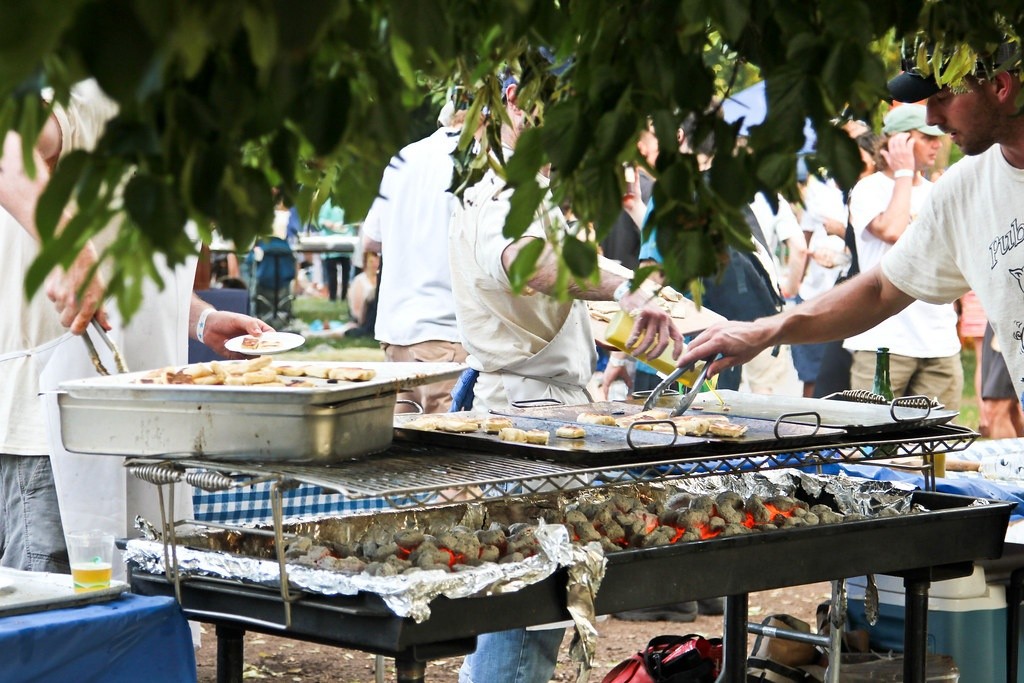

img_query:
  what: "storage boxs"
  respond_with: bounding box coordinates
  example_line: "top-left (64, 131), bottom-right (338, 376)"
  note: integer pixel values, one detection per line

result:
top-left (842, 565), bottom-right (1024, 683)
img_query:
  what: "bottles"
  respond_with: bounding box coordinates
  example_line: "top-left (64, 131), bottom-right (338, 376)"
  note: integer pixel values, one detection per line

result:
top-left (604, 310), bottom-right (709, 389)
top-left (866, 347), bottom-right (897, 458)
top-left (923, 400), bottom-right (945, 480)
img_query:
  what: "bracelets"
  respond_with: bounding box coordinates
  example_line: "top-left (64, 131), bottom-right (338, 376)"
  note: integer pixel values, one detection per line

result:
top-left (195, 308), bottom-right (217, 345)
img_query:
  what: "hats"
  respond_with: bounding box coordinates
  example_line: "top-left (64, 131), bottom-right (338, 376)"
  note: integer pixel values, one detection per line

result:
top-left (501, 47), bottom-right (577, 104)
top-left (888, 43), bottom-right (1024, 103)
top-left (882, 103), bottom-right (947, 136)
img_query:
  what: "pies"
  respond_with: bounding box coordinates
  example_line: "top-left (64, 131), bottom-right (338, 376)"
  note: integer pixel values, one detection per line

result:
top-left (240, 336), bottom-right (282, 349)
top-left (138, 355), bottom-right (377, 389)
top-left (401, 408), bottom-right (743, 444)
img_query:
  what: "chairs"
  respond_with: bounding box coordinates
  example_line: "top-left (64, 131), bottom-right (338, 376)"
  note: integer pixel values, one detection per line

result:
top-left (186, 288), bottom-right (251, 362)
top-left (249, 250), bottom-right (298, 319)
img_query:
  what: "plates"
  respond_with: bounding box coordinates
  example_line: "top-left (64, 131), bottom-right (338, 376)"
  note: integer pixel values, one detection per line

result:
top-left (224, 332), bottom-right (306, 356)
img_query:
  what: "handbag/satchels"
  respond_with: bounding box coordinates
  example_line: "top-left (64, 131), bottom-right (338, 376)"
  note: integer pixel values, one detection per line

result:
top-left (694, 244), bottom-right (787, 358)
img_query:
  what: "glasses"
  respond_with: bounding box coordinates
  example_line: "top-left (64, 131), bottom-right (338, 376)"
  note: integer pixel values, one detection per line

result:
top-left (901, 56), bottom-right (1000, 72)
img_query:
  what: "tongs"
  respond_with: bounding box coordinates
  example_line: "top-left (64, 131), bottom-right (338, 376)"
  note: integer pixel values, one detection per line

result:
top-left (81, 316), bottom-right (130, 376)
top-left (830, 571), bottom-right (879, 629)
top-left (641, 353), bottom-right (719, 418)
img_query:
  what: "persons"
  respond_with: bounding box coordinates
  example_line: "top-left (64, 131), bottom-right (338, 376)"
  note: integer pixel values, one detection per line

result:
top-left (216, 199), bottom-right (381, 328)
top-left (354, 69), bottom-right (492, 414)
top-left (0, 79), bottom-right (277, 579)
top-left (443, 48), bottom-right (1023, 682)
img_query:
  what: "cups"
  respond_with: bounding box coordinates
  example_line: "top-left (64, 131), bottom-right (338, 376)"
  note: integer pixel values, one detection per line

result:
top-left (66, 533), bottom-right (114, 592)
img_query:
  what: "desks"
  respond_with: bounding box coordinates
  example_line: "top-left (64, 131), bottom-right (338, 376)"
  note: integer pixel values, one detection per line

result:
top-left (205, 238), bottom-right (358, 306)
top-left (0, 565), bottom-right (197, 683)
